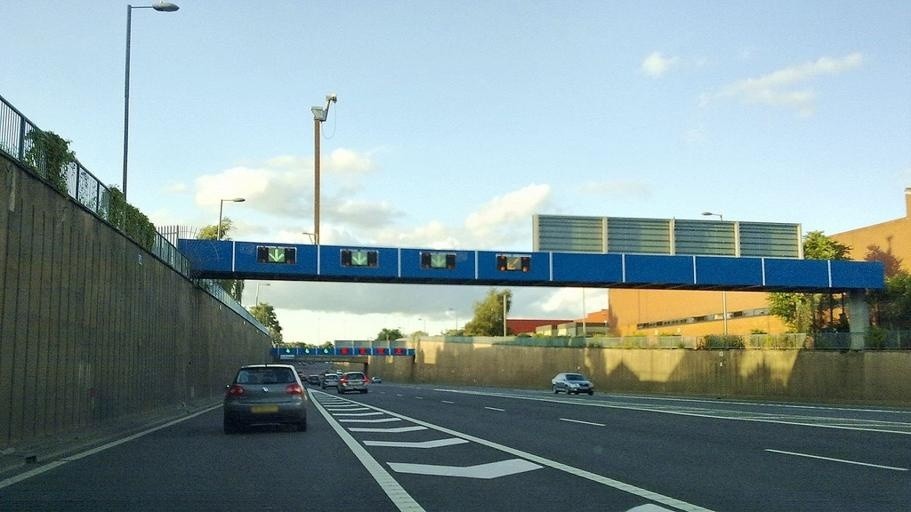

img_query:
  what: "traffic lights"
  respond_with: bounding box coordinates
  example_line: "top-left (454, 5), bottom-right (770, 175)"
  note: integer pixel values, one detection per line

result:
top-left (419, 250), bottom-right (454, 267)
top-left (341, 249), bottom-right (376, 267)
top-left (498, 254), bottom-right (532, 272)
top-left (258, 245), bottom-right (295, 264)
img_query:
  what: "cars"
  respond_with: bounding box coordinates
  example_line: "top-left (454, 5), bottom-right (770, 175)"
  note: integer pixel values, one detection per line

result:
top-left (298, 369), bottom-right (381, 394)
top-left (551, 372), bottom-right (595, 396)
top-left (224, 364), bottom-right (307, 432)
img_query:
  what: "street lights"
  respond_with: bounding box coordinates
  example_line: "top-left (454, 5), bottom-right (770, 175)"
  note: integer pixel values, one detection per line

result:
top-left (217, 198), bottom-right (245, 241)
top-left (122, 2), bottom-right (179, 200)
top-left (703, 211), bottom-right (727, 336)
